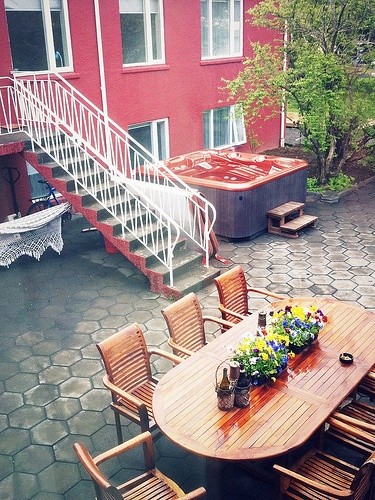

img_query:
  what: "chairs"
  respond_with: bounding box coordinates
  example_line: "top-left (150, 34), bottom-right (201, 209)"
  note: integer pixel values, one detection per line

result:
top-left (73, 265), bottom-right (375, 500)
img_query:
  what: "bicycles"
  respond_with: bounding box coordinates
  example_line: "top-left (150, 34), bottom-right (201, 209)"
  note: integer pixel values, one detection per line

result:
top-left (27, 179), bottom-right (72, 222)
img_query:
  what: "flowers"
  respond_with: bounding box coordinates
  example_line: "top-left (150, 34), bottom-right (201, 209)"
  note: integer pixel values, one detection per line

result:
top-left (231, 303), bottom-right (329, 385)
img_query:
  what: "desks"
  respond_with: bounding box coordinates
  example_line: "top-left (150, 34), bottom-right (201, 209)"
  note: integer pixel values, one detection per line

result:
top-left (151, 297), bottom-right (375, 464)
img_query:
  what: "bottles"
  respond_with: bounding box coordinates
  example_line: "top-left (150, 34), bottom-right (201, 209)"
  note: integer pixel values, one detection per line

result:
top-left (235, 364), bottom-right (248, 387)
top-left (219, 368), bottom-right (230, 390)
top-left (256, 311), bottom-right (267, 336)
top-left (229, 358), bottom-right (239, 382)
